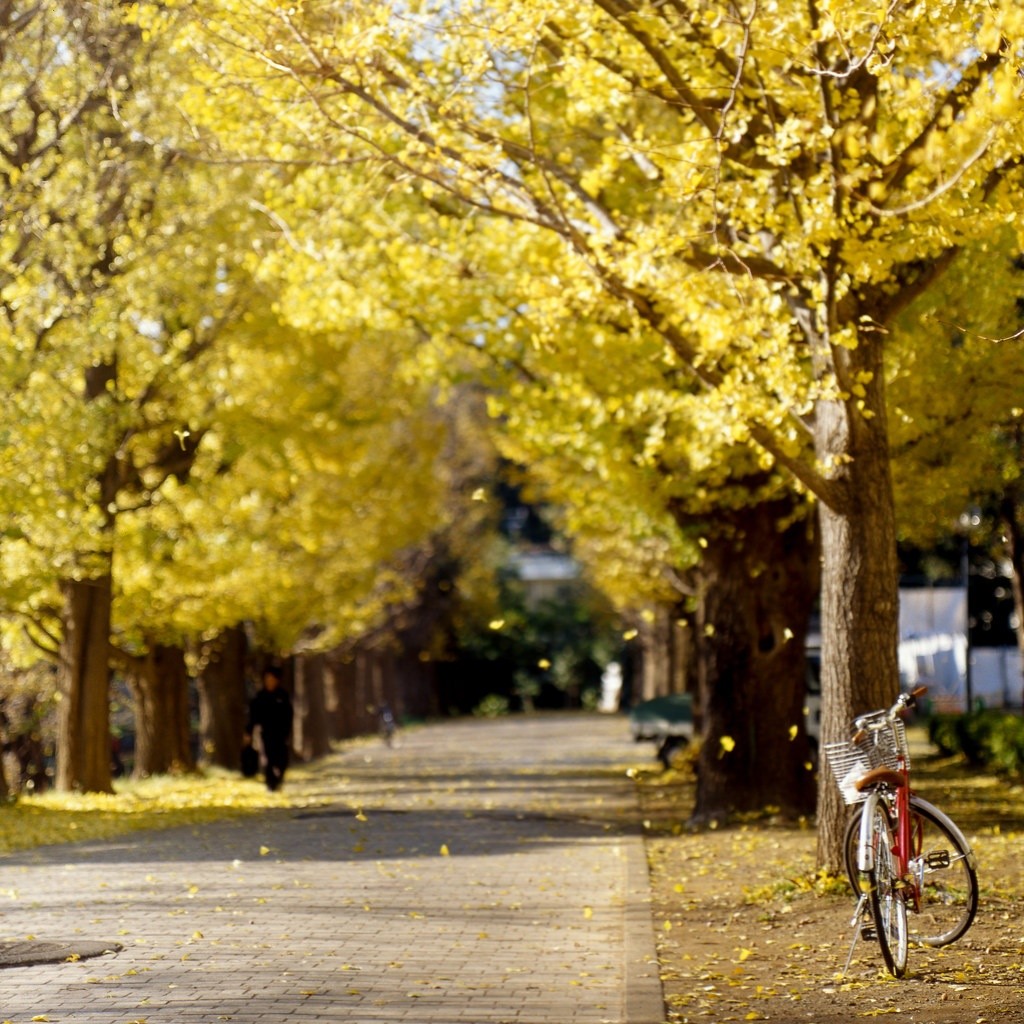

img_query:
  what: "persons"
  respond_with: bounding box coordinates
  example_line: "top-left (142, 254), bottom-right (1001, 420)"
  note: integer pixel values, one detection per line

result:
top-left (243, 669), bottom-right (294, 791)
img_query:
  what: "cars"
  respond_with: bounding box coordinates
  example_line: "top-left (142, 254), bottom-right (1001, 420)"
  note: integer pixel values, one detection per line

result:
top-left (630, 648), bottom-right (828, 772)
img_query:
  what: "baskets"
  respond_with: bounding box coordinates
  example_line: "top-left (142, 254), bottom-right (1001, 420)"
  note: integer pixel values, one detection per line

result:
top-left (822, 708), bottom-right (910, 805)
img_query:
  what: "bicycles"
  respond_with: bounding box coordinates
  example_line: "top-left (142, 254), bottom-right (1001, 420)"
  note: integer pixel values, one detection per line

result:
top-left (824, 685), bottom-right (979, 978)
